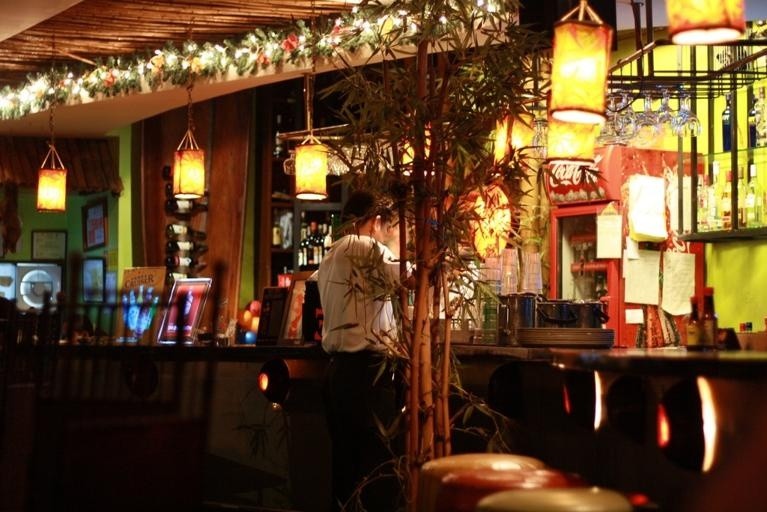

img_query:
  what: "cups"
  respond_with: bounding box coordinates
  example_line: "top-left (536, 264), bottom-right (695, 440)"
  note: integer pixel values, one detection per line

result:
top-left (485, 250), bottom-right (541, 293)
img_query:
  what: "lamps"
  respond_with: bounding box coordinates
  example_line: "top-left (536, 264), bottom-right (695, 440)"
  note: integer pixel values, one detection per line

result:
top-left (548, 0), bottom-right (614, 124)
top-left (561, 370), bottom-right (603, 431)
top-left (172, 12), bottom-right (205, 201)
top-left (653, 376), bottom-right (717, 472)
top-left (292, 10), bottom-right (330, 200)
top-left (36, 29), bottom-right (68, 213)
top-left (257, 360), bottom-right (289, 405)
top-left (665, 0), bottom-right (746, 45)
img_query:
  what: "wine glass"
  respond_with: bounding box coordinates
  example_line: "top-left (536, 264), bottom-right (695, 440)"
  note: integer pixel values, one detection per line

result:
top-left (600, 84), bottom-right (702, 140)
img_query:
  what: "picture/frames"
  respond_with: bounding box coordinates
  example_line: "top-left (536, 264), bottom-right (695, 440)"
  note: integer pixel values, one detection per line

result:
top-left (156, 277), bottom-right (213, 344)
top-left (81, 195), bottom-right (109, 251)
top-left (30, 230), bottom-right (68, 261)
top-left (82, 257), bottom-right (107, 304)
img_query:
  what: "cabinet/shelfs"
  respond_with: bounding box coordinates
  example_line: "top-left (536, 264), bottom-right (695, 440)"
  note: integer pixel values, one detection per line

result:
top-left (677, 44), bottom-right (767, 243)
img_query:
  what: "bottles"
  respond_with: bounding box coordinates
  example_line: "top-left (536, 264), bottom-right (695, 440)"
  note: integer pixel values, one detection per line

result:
top-left (273, 207), bottom-right (335, 270)
top-left (122, 285), bottom-right (159, 339)
top-left (688, 288), bottom-right (717, 350)
top-left (739, 322), bottom-right (751, 334)
top-left (164, 185), bottom-right (208, 283)
top-left (697, 164), bottom-right (761, 230)
top-left (722, 86), bottom-right (767, 152)
top-left (594, 275), bottom-right (605, 299)
top-left (184, 289), bottom-right (194, 322)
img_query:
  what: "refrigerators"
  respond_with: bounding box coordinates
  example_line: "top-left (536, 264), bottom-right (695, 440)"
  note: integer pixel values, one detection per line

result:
top-left (542, 145), bottom-right (705, 348)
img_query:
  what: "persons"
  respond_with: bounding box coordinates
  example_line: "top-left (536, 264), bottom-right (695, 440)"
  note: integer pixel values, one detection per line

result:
top-left (318, 190), bottom-right (436, 489)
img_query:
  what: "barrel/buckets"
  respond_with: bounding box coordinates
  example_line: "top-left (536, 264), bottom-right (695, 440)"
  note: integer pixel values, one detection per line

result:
top-left (537, 301), bottom-right (577, 328)
top-left (570, 304), bottom-right (609, 328)
top-left (498, 294), bottom-right (534, 345)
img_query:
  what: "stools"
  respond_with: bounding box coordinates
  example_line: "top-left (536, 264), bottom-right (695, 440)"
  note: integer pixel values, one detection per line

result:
top-left (415, 454), bottom-right (633, 512)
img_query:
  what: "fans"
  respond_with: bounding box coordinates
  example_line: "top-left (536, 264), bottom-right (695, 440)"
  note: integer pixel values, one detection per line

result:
top-left (277, 271), bottom-right (316, 347)
top-left (256, 286), bottom-right (288, 344)
top-left (15, 263), bottom-right (62, 313)
